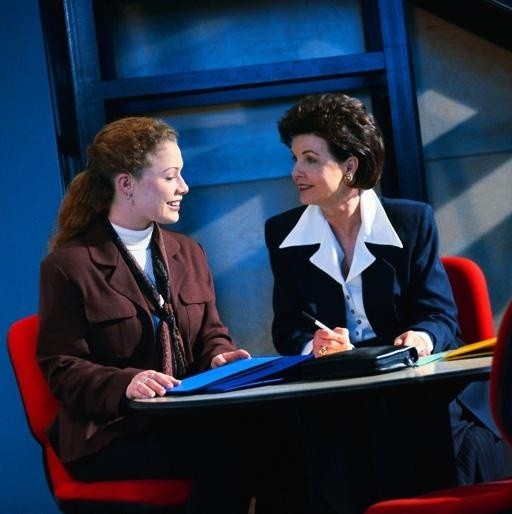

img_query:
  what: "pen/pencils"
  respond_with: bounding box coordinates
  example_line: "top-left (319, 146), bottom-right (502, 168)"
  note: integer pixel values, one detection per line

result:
top-left (303, 311), bottom-right (334, 331)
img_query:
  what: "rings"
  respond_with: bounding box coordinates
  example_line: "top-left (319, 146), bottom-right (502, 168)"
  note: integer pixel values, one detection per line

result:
top-left (318, 345), bottom-right (328, 355)
top-left (142, 376), bottom-right (150, 382)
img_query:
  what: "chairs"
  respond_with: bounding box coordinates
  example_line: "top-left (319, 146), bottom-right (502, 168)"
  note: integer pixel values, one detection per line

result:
top-left (8, 313), bottom-right (191, 514)
top-left (362, 254), bottom-right (512, 513)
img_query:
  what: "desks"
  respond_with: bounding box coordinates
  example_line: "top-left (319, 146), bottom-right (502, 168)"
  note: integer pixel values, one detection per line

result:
top-left (130, 352), bottom-right (492, 513)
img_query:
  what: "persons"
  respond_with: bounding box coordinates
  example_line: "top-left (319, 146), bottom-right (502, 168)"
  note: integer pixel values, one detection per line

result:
top-left (262, 92), bottom-right (506, 513)
top-left (34, 115), bottom-right (253, 510)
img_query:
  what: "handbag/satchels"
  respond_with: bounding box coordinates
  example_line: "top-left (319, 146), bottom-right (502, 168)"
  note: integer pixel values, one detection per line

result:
top-left (297, 345), bottom-right (419, 382)
